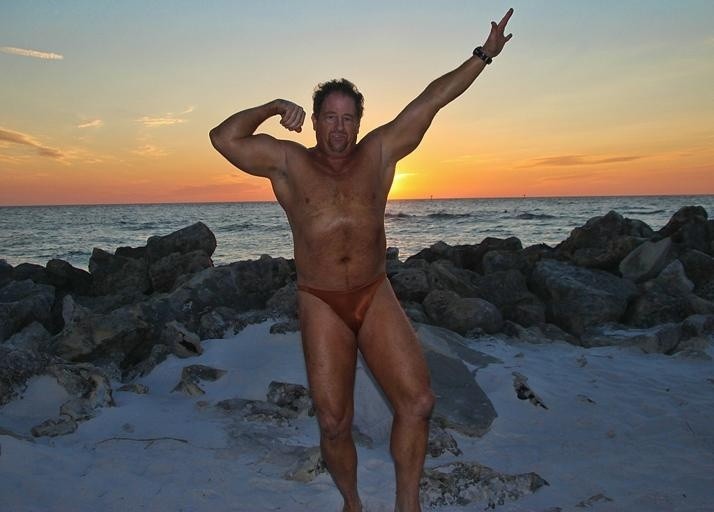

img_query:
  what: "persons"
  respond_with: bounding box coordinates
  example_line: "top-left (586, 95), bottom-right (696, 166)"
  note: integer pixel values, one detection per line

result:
top-left (209, 7), bottom-right (514, 511)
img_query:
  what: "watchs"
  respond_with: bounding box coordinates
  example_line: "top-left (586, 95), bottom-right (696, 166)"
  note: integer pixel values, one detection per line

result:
top-left (472, 46), bottom-right (493, 67)
